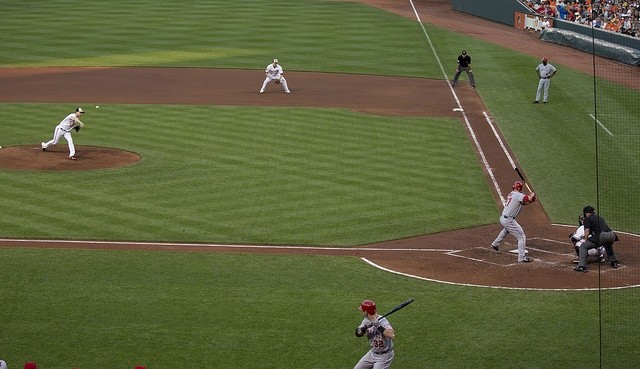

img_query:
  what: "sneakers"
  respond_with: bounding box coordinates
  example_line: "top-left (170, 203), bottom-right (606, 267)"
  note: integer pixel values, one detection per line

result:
top-left (521, 256), bottom-right (532, 262)
top-left (574, 266), bottom-right (588, 273)
top-left (599, 256), bottom-right (606, 262)
top-left (71, 156), bottom-right (76, 160)
top-left (43, 148), bottom-right (46, 151)
top-left (491, 243), bottom-right (501, 253)
top-left (260, 89), bottom-right (264, 93)
top-left (573, 259), bottom-right (579, 264)
top-left (611, 261), bottom-right (618, 268)
top-left (285, 89), bottom-right (290, 94)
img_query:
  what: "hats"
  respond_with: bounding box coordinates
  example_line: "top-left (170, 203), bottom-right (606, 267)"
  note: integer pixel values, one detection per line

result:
top-left (272, 58), bottom-right (278, 63)
top-left (541, 57), bottom-right (548, 62)
top-left (462, 51), bottom-right (466, 55)
top-left (75, 108), bottom-right (84, 112)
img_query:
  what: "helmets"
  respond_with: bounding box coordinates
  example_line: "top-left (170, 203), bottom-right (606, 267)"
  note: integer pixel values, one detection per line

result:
top-left (512, 181), bottom-right (523, 190)
top-left (583, 205), bottom-right (595, 218)
top-left (357, 299), bottom-right (376, 314)
top-left (578, 215), bottom-right (586, 226)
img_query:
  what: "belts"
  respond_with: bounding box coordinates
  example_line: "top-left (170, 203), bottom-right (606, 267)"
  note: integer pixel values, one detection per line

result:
top-left (374, 348), bottom-right (390, 355)
top-left (61, 127), bottom-right (66, 131)
top-left (504, 215), bottom-right (515, 219)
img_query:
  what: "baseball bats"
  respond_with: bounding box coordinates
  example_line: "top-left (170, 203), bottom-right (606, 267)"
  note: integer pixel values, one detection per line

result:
top-left (365, 298), bottom-right (415, 328)
top-left (514, 167), bottom-right (536, 201)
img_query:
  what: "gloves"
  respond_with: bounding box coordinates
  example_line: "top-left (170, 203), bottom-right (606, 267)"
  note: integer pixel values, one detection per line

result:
top-left (364, 320), bottom-right (373, 329)
top-left (372, 317), bottom-right (381, 328)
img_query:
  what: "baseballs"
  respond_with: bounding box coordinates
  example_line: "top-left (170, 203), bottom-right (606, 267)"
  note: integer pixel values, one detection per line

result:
top-left (95, 106), bottom-right (99, 109)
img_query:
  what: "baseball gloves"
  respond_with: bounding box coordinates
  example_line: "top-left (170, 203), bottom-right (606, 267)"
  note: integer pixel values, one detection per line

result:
top-left (74, 126), bottom-right (81, 133)
top-left (273, 78), bottom-right (281, 87)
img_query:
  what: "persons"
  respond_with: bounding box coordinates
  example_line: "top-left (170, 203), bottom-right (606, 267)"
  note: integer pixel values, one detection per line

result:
top-left (533, 57), bottom-right (557, 104)
top-left (569, 216), bottom-right (606, 264)
top-left (41, 108), bottom-right (85, 160)
top-left (526, 0), bottom-right (554, 16)
top-left (573, 205), bottom-right (618, 273)
top-left (491, 180), bottom-right (536, 263)
top-left (259, 59), bottom-right (291, 94)
top-left (554, 0), bottom-right (640, 40)
top-left (452, 51), bottom-right (476, 88)
top-left (352, 299), bottom-right (396, 369)
top-left (539, 16), bottom-right (550, 38)
